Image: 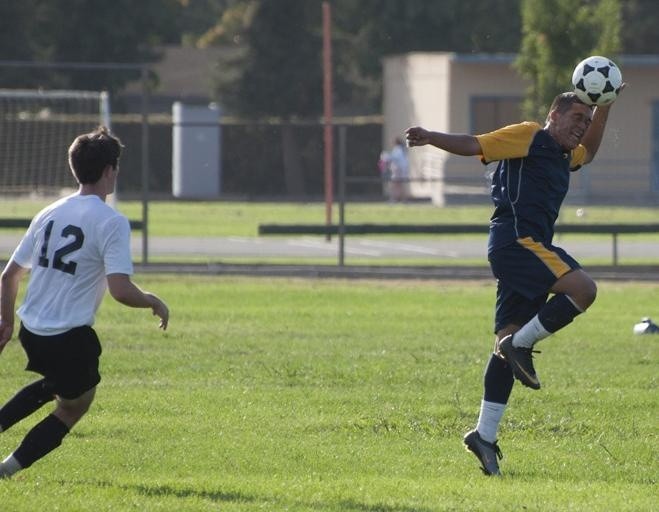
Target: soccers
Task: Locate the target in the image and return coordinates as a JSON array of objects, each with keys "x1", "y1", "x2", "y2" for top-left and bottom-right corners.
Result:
[{"x1": 572, "y1": 56, "x2": 622, "y2": 107}]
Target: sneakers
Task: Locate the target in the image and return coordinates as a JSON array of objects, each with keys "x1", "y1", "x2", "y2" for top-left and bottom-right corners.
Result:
[
  {"x1": 500, "y1": 334, "x2": 542, "y2": 391},
  {"x1": 463, "y1": 428, "x2": 501, "y2": 478}
]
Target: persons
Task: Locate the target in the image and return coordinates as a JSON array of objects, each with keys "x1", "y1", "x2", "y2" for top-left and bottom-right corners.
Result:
[
  {"x1": 385, "y1": 135, "x2": 410, "y2": 204},
  {"x1": 0, "y1": 126, "x2": 169, "y2": 481},
  {"x1": 404, "y1": 81, "x2": 628, "y2": 475}
]
